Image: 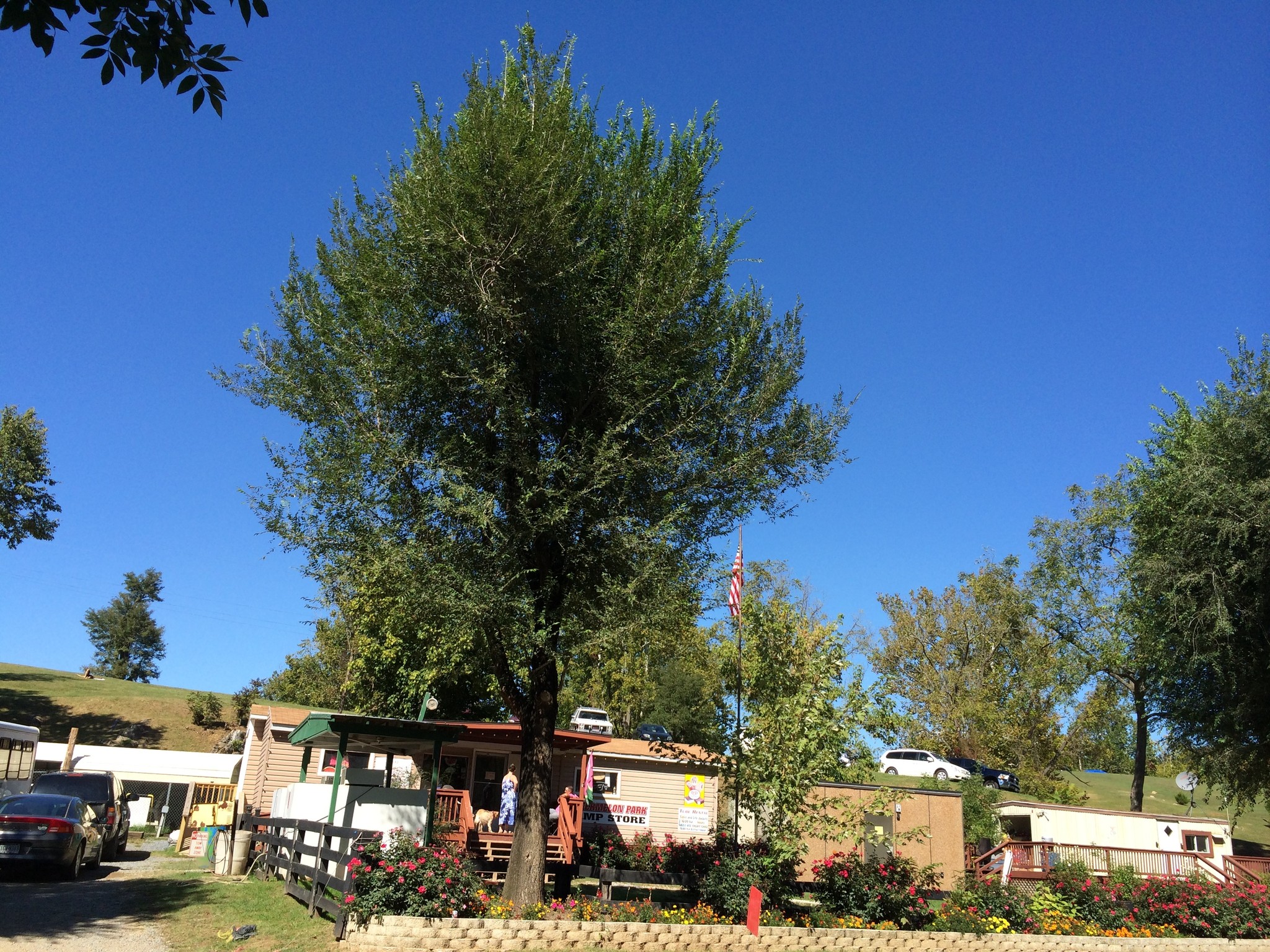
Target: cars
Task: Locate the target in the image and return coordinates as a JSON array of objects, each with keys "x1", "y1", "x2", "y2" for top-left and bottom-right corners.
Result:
[
  {"x1": 0, "y1": 793, "x2": 109, "y2": 882},
  {"x1": 631, "y1": 723, "x2": 673, "y2": 743}
]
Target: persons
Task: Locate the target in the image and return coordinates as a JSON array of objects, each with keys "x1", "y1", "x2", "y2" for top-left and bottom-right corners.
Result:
[
  {"x1": 75, "y1": 669, "x2": 103, "y2": 679},
  {"x1": 498, "y1": 764, "x2": 519, "y2": 834},
  {"x1": 549, "y1": 786, "x2": 579, "y2": 834}
]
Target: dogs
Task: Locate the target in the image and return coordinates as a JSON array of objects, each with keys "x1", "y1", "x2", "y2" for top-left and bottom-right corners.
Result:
[{"x1": 473, "y1": 808, "x2": 500, "y2": 833}]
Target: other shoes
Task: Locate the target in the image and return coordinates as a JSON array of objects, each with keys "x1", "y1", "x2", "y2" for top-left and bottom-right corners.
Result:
[
  {"x1": 503, "y1": 830, "x2": 513, "y2": 834},
  {"x1": 498, "y1": 830, "x2": 504, "y2": 833}
]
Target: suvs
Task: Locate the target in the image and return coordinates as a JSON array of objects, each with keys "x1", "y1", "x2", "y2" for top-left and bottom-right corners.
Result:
[
  {"x1": 878, "y1": 748, "x2": 971, "y2": 783},
  {"x1": 946, "y1": 757, "x2": 1020, "y2": 794},
  {"x1": 28, "y1": 770, "x2": 139, "y2": 862},
  {"x1": 569, "y1": 707, "x2": 613, "y2": 736}
]
